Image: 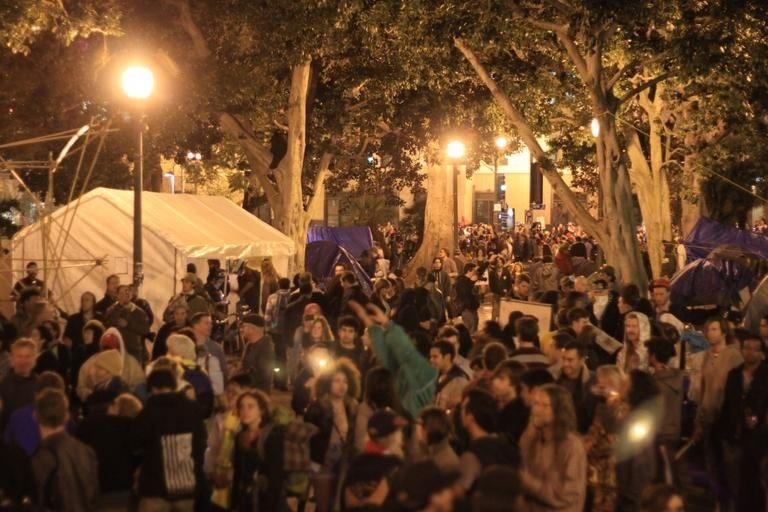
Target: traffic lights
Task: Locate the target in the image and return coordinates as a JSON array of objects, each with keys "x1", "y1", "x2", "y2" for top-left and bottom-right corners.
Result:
[{"x1": 496, "y1": 174, "x2": 505, "y2": 202}]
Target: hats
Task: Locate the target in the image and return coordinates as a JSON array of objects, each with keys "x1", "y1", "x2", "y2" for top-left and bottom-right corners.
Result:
[
  {"x1": 232, "y1": 259, "x2": 244, "y2": 274},
  {"x1": 95, "y1": 349, "x2": 123, "y2": 378},
  {"x1": 366, "y1": 408, "x2": 407, "y2": 439},
  {"x1": 649, "y1": 278, "x2": 669, "y2": 292},
  {"x1": 181, "y1": 272, "x2": 197, "y2": 284},
  {"x1": 208, "y1": 268, "x2": 224, "y2": 278},
  {"x1": 239, "y1": 314, "x2": 266, "y2": 329},
  {"x1": 299, "y1": 272, "x2": 312, "y2": 286},
  {"x1": 166, "y1": 333, "x2": 197, "y2": 361},
  {"x1": 598, "y1": 263, "x2": 616, "y2": 281}
]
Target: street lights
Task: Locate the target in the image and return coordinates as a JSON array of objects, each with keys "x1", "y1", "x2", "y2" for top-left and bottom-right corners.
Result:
[
  {"x1": 117, "y1": 64, "x2": 160, "y2": 286},
  {"x1": 184, "y1": 150, "x2": 202, "y2": 193},
  {"x1": 162, "y1": 169, "x2": 176, "y2": 194},
  {"x1": 491, "y1": 137, "x2": 506, "y2": 206},
  {"x1": 445, "y1": 139, "x2": 463, "y2": 253}
]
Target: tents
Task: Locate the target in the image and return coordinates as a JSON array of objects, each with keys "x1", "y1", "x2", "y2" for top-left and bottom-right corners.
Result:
[{"x1": 10, "y1": 188, "x2": 300, "y2": 335}]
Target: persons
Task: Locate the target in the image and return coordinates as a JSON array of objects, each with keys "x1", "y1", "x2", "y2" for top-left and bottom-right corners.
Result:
[{"x1": 1, "y1": 200, "x2": 767, "y2": 510}]
